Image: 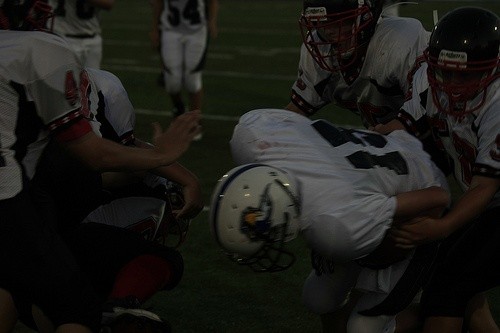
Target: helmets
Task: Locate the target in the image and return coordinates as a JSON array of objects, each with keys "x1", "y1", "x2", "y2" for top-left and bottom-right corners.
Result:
[
  {"x1": 208, "y1": 161, "x2": 301, "y2": 256},
  {"x1": 423, "y1": 5, "x2": 500, "y2": 86},
  {"x1": 104, "y1": 164, "x2": 191, "y2": 248},
  {"x1": 0, "y1": 0, "x2": 57, "y2": 33},
  {"x1": 298, "y1": 0, "x2": 383, "y2": 49}
]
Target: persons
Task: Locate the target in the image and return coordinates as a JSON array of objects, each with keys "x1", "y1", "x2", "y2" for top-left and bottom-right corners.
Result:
[
  {"x1": 284, "y1": 0, "x2": 430, "y2": 333},
  {"x1": 0, "y1": 0, "x2": 204, "y2": 333},
  {"x1": 209, "y1": 108, "x2": 451, "y2": 333},
  {"x1": 367, "y1": 6, "x2": 499, "y2": 333},
  {"x1": 152, "y1": 0, "x2": 218, "y2": 119}
]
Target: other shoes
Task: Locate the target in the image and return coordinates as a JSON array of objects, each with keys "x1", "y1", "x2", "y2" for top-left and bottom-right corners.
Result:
[{"x1": 101, "y1": 294, "x2": 170, "y2": 328}]
